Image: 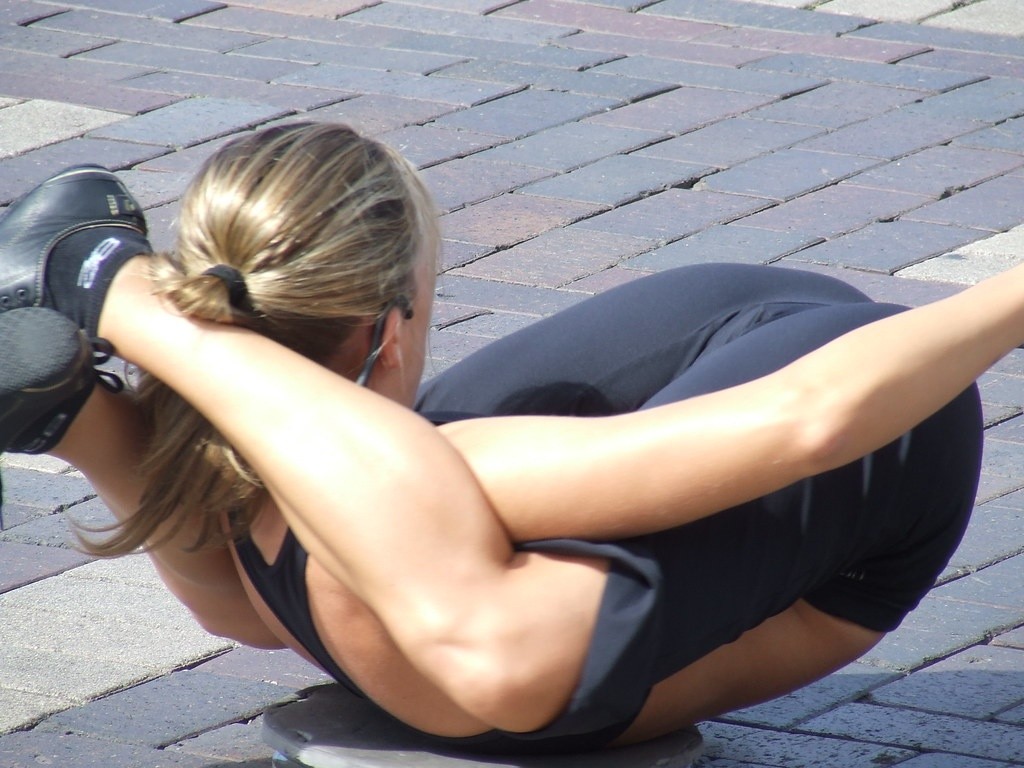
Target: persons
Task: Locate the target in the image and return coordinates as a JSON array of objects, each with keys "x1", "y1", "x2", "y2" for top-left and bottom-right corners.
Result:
[{"x1": 1, "y1": 123, "x2": 1024, "y2": 742}]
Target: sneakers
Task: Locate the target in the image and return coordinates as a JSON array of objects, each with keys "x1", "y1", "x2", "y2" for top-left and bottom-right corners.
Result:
[
  {"x1": 0, "y1": 164, "x2": 146, "y2": 312},
  {"x1": 0, "y1": 307, "x2": 97, "y2": 452}
]
